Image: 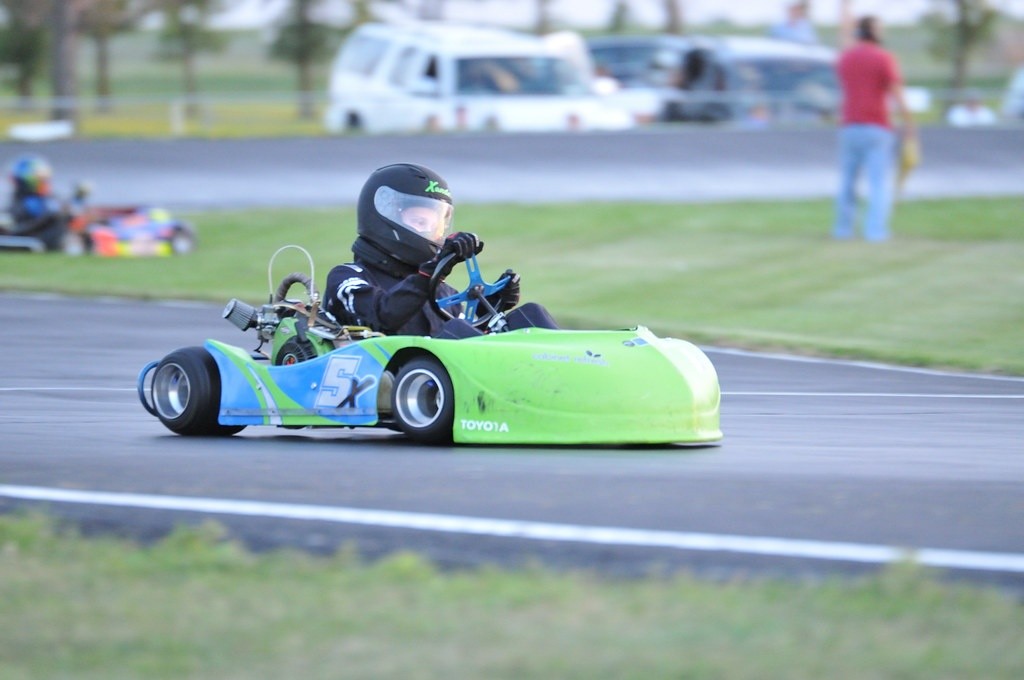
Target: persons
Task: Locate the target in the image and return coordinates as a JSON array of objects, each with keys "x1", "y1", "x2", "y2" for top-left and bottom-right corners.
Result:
[
  {"x1": 11, "y1": 155, "x2": 86, "y2": 252},
  {"x1": 656, "y1": 45, "x2": 730, "y2": 125},
  {"x1": 831, "y1": 16, "x2": 918, "y2": 243},
  {"x1": 325, "y1": 163, "x2": 520, "y2": 336}
]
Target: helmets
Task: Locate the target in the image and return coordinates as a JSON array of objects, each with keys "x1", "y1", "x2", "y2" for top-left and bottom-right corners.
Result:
[
  {"x1": 11, "y1": 156, "x2": 54, "y2": 196},
  {"x1": 352, "y1": 164, "x2": 453, "y2": 278}
]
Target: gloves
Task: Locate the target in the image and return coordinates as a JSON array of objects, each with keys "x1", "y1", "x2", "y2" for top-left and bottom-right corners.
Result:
[
  {"x1": 75, "y1": 187, "x2": 90, "y2": 198},
  {"x1": 419, "y1": 231, "x2": 484, "y2": 283},
  {"x1": 475, "y1": 269, "x2": 520, "y2": 327}
]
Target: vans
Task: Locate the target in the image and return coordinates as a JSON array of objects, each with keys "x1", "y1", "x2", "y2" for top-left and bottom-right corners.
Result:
[
  {"x1": 325, "y1": 19, "x2": 627, "y2": 133},
  {"x1": 590, "y1": 34, "x2": 854, "y2": 129}
]
